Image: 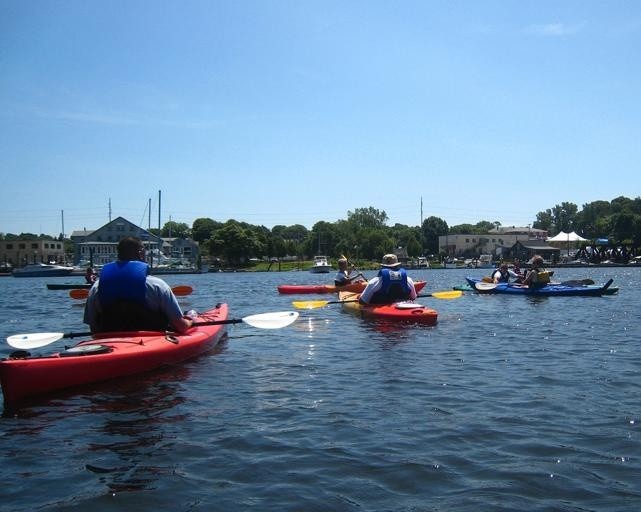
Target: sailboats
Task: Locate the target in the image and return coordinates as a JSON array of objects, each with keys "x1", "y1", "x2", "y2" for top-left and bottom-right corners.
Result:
[{"x1": 94, "y1": 186, "x2": 210, "y2": 276}]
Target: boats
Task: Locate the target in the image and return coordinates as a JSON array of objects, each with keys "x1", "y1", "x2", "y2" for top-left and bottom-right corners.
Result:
[
  {"x1": 453, "y1": 276, "x2": 619, "y2": 297},
  {"x1": 0, "y1": 302, "x2": 228, "y2": 405},
  {"x1": 12, "y1": 261, "x2": 74, "y2": 278},
  {"x1": 47, "y1": 283, "x2": 92, "y2": 290},
  {"x1": 277, "y1": 280, "x2": 438, "y2": 325},
  {"x1": 312, "y1": 256, "x2": 332, "y2": 273}
]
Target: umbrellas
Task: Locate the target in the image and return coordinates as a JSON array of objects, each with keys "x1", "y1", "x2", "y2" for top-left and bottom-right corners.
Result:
[{"x1": 545, "y1": 230, "x2": 591, "y2": 256}]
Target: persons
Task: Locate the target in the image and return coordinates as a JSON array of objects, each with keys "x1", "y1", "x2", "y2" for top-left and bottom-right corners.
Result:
[
  {"x1": 334, "y1": 259, "x2": 362, "y2": 286},
  {"x1": 83, "y1": 236, "x2": 193, "y2": 333},
  {"x1": 493, "y1": 263, "x2": 524, "y2": 283},
  {"x1": 85, "y1": 267, "x2": 96, "y2": 284},
  {"x1": 520, "y1": 255, "x2": 548, "y2": 285},
  {"x1": 356, "y1": 253, "x2": 417, "y2": 305}
]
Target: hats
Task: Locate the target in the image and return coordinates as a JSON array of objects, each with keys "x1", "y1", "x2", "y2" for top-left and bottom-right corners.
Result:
[{"x1": 379, "y1": 254, "x2": 404, "y2": 267}]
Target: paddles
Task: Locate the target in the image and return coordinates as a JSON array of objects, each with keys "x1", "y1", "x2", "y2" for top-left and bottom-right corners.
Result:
[
  {"x1": 69, "y1": 286, "x2": 193, "y2": 299},
  {"x1": 291, "y1": 291, "x2": 463, "y2": 309},
  {"x1": 6, "y1": 311, "x2": 299, "y2": 350},
  {"x1": 475, "y1": 277, "x2": 595, "y2": 290}
]
